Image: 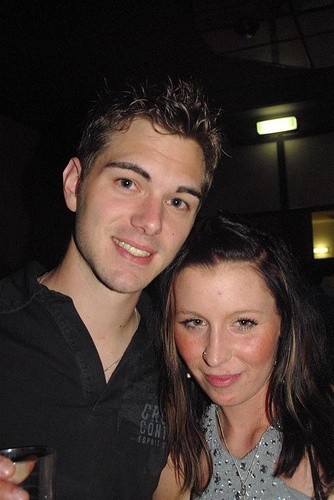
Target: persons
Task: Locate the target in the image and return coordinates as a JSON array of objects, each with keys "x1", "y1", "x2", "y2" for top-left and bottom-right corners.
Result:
[
  {"x1": 0, "y1": 74, "x2": 219, "y2": 500},
  {"x1": 151, "y1": 212, "x2": 334, "y2": 500}
]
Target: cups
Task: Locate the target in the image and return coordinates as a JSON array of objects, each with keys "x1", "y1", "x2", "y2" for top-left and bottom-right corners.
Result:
[{"x1": 0, "y1": 445, "x2": 57, "y2": 500}]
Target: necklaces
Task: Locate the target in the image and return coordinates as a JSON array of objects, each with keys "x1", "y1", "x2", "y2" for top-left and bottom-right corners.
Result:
[
  {"x1": 215, "y1": 405, "x2": 278, "y2": 500},
  {"x1": 39, "y1": 270, "x2": 139, "y2": 374}
]
